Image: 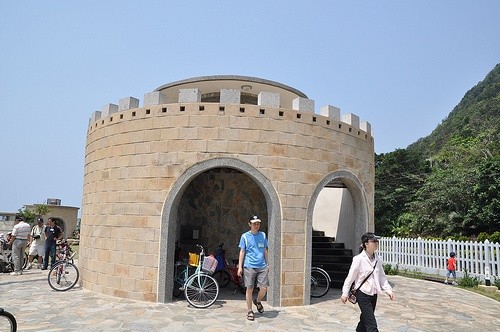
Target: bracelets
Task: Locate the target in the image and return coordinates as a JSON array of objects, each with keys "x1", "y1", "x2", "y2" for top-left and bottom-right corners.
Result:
[{"x1": 266, "y1": 263, "x2": 269, "y2": 266}]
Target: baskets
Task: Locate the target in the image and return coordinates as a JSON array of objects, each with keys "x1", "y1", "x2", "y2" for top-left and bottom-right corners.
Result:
[{"x1": 202, "y1": 255, "x2": 218, "y2": 275}]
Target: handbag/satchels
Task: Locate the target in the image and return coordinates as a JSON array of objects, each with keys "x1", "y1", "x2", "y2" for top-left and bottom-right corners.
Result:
[{"x1": 348, "y1": 289, "x2": 357, "y2": 304}]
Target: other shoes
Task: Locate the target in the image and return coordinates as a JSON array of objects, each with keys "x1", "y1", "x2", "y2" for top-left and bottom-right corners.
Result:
[
  {"x1": 452, "y1": 281, "x2": 459, "y2": 285},
  {"x1": 41, "y1": 265, "x2": 47, "y2": 270},
  {"x1": 25, "y1": 265, "x2": 33, "y2": 270},
  {"x1": 445, "y1": 281, "x2": 449, "y2": 284},
  {"x1": 10, "y1": 271, "x2": 22, "y2": 275}
]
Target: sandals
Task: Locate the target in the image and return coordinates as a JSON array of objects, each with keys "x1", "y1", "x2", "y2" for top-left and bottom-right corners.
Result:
[
  {"x1": 253, "y1": 298, "x2": 264, "y2": 313},
  {"x1": 247, "y1": 311, "x2": 254, "y2": 320}
]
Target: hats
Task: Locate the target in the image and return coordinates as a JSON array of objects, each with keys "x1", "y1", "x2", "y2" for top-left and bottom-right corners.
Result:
[
  {"x1": 362, "y1": 232, "x2": 381, "y2": 243},
  {"x1": 450, "y1": 252, "x2": 455, "y2": 257},
  {"x1": 249, "y1": 215, "x2": 262, "y2": 223},
  {"x1": 36, "y1": 217, "x2": 43, "y2": 223}
]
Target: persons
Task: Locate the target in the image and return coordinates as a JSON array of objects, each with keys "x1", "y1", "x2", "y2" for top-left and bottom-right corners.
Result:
[
  {"x1": 0, "y1": 232, "x2": 12, "y2": 250},
  {"x1": 72, "y1": 230, "x2": 76, "y2": 236},
  {"x1": 25, "y1": 218, "x2": 46, "y2": 270},
  {"x1": 237, "y1": 214, "x2": 269, "y2": 320},
  {"x1": 6, "y1": 215, "x2": 31, "y2": 276},
  {"x1": 41, "y1": 217, "x2": 65, "y2": 270},
  {"x1": 341, "y1": 232, "x2": 395, "y2": 332},
  {"x1": 444, "y1": 252, "x2": 458, "y2": 285}
]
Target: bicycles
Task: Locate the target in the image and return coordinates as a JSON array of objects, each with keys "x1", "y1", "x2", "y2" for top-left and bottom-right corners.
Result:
[
  {"x1": 174, "y1": 239, "x2": 246, "y2": 309},
  {"x1": 310, "y1": 264, "x2": 332, "y2": 299},
  {"x1": 47, "y1": 237, "x2": 79, "y2": 292},
  {"x1": 0, "y1": 238, "x2": 28, "y2": 272}
]
